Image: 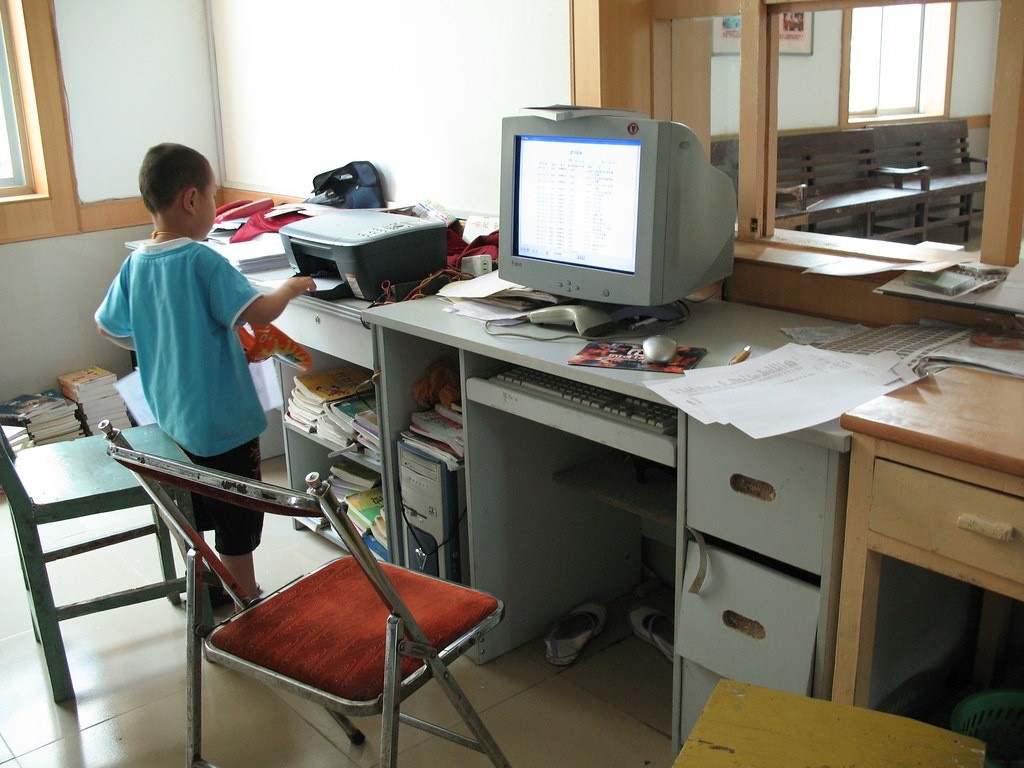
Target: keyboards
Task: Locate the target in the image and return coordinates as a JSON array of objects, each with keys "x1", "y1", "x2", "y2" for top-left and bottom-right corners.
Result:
[{"x1": 489, "y1": 367, "x2": 678, "y2": 434}]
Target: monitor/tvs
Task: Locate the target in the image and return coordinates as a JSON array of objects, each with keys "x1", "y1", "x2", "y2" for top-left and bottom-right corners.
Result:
[{"x1": 498, "y1": 114, "x2": 738, "y2": 339}]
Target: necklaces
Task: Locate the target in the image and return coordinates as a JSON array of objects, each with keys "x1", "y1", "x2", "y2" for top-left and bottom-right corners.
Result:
[{"x1": 152, "y1": 231, "x2": 182, "y2": 239}]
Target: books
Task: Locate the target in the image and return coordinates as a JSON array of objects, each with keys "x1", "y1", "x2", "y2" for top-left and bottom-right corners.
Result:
[
  {"x1": 284, "y1": 367, "x2": 388, "y2": 561},
  {"x1": 400, "y1": 402, "x2": 464, "y2": 472},
  {"x1": 0, "y1": 366, "x2": 132, "y2": 501},
  {"x1": 437, "y1": 279, "x2": 574, "y2": 313}
]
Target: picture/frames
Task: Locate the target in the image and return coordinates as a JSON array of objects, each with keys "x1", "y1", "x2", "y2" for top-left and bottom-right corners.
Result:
[{"x1": 711, "y1": 11, "x2": 815, "y2": 57}]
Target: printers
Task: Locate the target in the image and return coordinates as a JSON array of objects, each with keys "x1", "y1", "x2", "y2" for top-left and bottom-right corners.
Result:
[{"x1": 279, "y1": 209, "x2": 448, "y2": 302}]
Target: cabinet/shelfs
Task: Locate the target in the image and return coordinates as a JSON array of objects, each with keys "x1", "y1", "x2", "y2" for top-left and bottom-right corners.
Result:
[
  {"x1": 830, "y1": 338, "x2": 1024, "y2": 709},
  {"x1": 359, "y1": 285, "x2": 949, "y2": 768},
  {"x1": 124, "y1": 237, "x2": 394, "y2": 568}
]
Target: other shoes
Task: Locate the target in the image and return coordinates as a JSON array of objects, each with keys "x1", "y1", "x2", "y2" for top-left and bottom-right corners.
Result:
[
  {"x1": 543, "y1": 601, "x2": 607, "y2": 666},
  {"x1": 209, "y1": 580, "x2": 260, "y2": 607},
  {"x1": 630, "y1": 607, "x2": 673, "y2": 662}
]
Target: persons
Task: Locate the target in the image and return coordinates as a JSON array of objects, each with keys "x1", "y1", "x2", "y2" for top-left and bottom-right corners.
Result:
[{"x1": 95, "y1": 142, "x2": 317, "y2": 615}]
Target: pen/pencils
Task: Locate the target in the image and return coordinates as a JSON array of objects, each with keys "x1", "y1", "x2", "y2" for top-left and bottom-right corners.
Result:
[
  {"x1": 628, "y1": 317, "x2": 658, "y2": 331},
  {"x1": 725, "y1": 344, "x2": 751, "y2": 369}
]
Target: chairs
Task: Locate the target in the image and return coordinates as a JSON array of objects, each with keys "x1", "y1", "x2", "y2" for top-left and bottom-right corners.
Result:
[
  {"x1": 100, "y1": 419, "x2": 508, "y2": 768},
  {"x1": 0, "y1": 419, "x2": 215, "y2": 705},
  {"x1": 670, "y1": 679, "x2": 987, "y2": 768}
]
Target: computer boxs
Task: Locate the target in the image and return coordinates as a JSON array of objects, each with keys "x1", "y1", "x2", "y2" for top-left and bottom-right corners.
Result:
[{"x1": 396, "y1": 441, "x2": 470, "y2": 587}]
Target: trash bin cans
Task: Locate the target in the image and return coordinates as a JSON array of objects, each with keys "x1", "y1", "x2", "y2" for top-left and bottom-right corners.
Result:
[{"x1": 949, "y1": 690, "x2": 1024, "y2": 768}]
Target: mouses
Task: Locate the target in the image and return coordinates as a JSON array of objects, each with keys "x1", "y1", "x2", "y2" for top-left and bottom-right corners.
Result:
[{"x1": 643, "y1": 334, "x2": 678, "y2": 364}]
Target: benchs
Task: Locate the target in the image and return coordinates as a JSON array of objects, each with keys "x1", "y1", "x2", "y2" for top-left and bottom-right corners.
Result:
[
  {"x1": 711, "y1": 128, "x2": 929, "y2": 245},
  {"x1": 866, "y1": 120, "x2": 988, "y2": 243}
]
